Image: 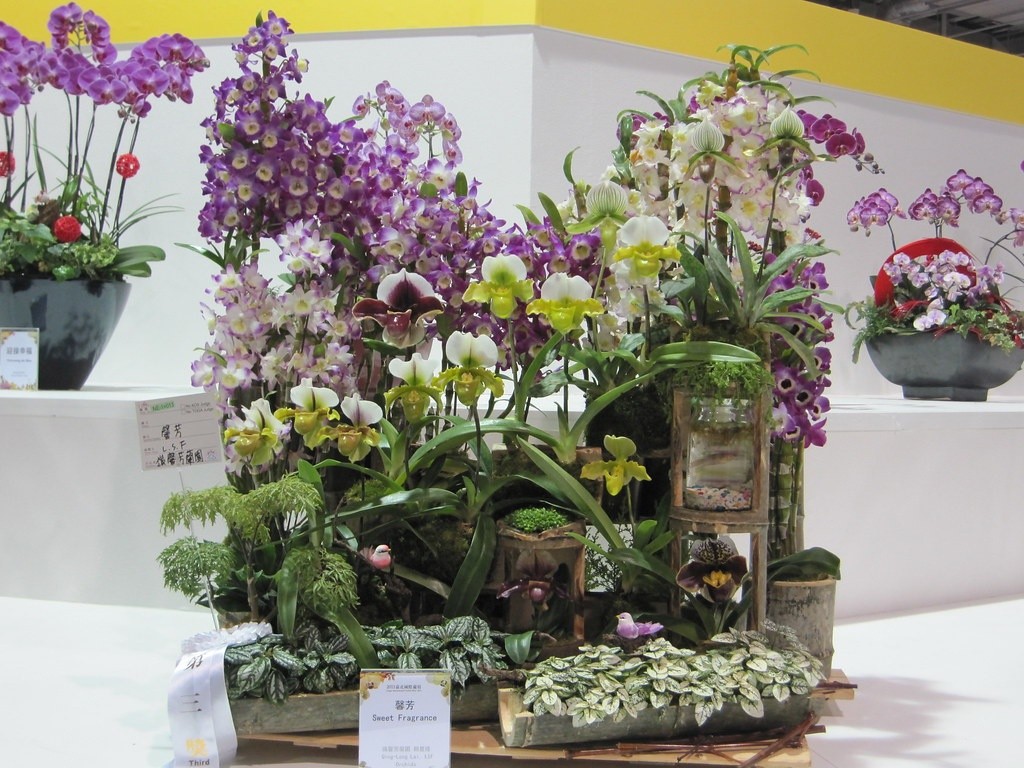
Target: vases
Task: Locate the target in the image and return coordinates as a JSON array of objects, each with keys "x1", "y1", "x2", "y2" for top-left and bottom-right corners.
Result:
[
  {"x1": 0, "y1": 276, "x2": 131, "y2": 389},
  {"x1": 863, "y1": 329, "x2": 1024, "y2": 402},
  {"x1": 742, "y1": 570, "x2": 837, "y2": 680}
]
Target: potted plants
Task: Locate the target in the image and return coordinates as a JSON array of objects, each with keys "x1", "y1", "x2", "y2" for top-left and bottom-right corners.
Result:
[
  {"x1": 494, "y1": 506, "x2": 588, "y2": 646},
  {"x1": 497, "y1": 637, "x2": 816, "y2": 755},
  {"x1": 220, "y1": 609, "x2": 512, "y2": 738}
]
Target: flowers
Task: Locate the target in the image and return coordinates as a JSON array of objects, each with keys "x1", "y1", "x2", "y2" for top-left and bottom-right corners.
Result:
[
  {"x1": 152, "y1": 4, "x2": 887, "y2": 610},
  {"x1": 0, "y1": 0, "x2": 211, "y2": 291},
  {"x1": 842, "y1": 167, "x2": 1024, "y2": 366}
]
